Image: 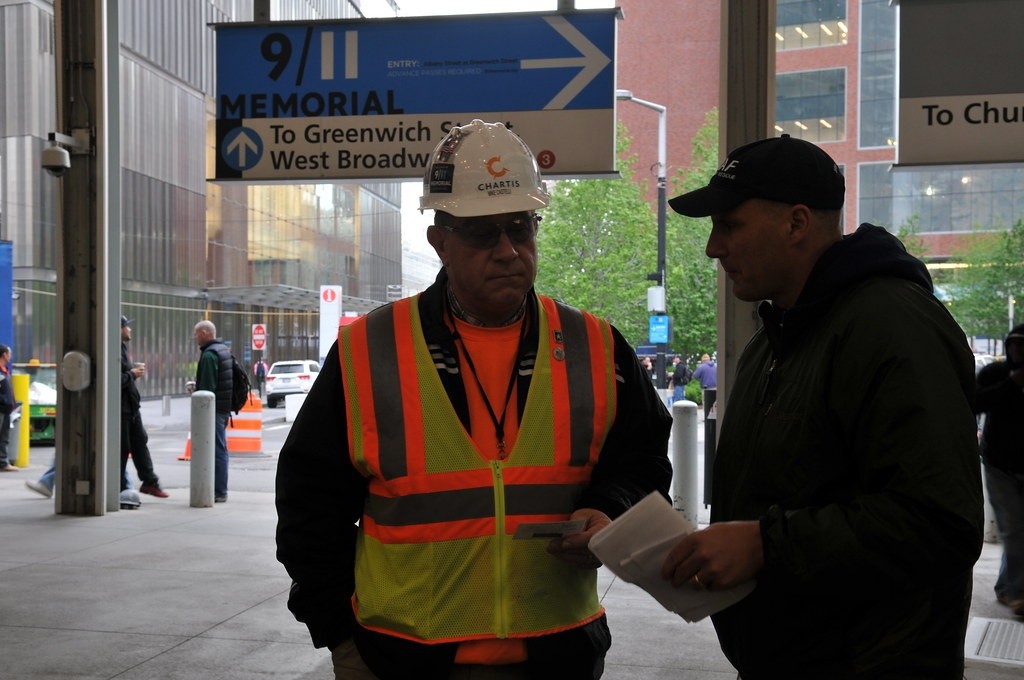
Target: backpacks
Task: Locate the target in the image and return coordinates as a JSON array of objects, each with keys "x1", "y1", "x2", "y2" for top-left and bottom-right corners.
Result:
[{"x1": 230, "y1": 354, "x2": 253, "y2": 415}]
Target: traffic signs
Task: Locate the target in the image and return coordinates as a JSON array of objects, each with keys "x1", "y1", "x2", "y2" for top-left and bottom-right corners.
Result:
[{"x1": 201, "y1": 6, "x2": 628, "y2": 188}]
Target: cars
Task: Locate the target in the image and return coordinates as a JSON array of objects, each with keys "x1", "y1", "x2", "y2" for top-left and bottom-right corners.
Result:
[{"x1": 9, "y1": 359, "x2": 56, "y2": 445}]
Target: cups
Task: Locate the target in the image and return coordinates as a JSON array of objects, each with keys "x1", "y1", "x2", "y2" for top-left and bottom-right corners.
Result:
[
  {"x1": 134, "y1": 363, "x2": 145, "y2": 376},
  {"x1": 186, "y1": 382, "x2": 196, "y2": 394}
]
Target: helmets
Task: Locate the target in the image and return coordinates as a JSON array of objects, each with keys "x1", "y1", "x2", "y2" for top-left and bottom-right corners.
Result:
[{"x1": 418, "y1": 117, "x2": 550, "y2": 220}]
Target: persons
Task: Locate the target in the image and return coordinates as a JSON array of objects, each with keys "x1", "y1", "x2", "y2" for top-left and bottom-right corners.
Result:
[
  {"x1": 671, "y1": 355, "x2": 686, "y2": 403},
  {"x1": 253, "y1": 357, "x2": 269, "y2": 398},
  {"x1": 660, "y1": 135, "x2": 983, "y2": 680},
  {"x1": 23, "y1": 462, "x2": 56, "y2": 497},
  {"x1": 641, "y1": 356, "x2": 652, "y2": 381},
  {"x1": 972, "y1": 324, "x2": 1024, "y2": 626},
  {"x1": 120, "y1": 314, "x2": 170, "y2": 498},
  {"x1": 272, "y1": 119, "x2": 673, "y2": 680},
  {"x1": 192, "y1": 319, "x2": 233, "y2": 502},
  {"x1": 0, "y1": 344, "x2": 20, "y2": 472},
  {"x1": 693, "y1": 353, "x2": 717, "y2": 422}
]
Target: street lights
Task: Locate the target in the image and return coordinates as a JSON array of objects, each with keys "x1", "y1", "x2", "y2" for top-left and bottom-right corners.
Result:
[{"x1": 616, "y1": 88, "x2": 671, "y2": 388}]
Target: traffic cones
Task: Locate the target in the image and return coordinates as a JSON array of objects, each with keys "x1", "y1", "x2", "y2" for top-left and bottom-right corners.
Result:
[{"x1": 175, "y1": 430, "x2": 192, "y2": 461}]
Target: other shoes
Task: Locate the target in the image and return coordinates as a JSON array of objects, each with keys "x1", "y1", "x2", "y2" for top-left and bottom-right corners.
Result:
[
  {"x1": 2, "y1": 463, "x2": 19, "y2": 471},
  {"x1": 25, "y1": 478, "x2": 53, "y2": 498}
]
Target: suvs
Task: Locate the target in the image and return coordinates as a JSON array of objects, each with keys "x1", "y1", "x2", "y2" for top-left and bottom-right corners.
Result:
[{"x1": 264, "y1": 359, "x2": 321, "y2": 408}]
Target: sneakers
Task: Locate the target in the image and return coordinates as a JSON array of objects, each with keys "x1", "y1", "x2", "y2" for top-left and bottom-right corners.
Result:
[
  {"x1": 214, "y1": 492, "x2": 228, "y2": 503},
  {"x1": 139, "y1": 483, "x2": 168, "y2": 497}
]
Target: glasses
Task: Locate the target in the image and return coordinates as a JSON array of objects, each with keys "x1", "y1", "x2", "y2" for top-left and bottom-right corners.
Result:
[{"x1": 438, "y1": 211, "x2": 538, "y2": 250}]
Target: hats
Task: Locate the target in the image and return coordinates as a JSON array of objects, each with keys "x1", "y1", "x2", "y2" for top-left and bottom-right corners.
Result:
[
  {"x1": 120, "y1": 315, "x2": 135, "y2": 328},
  {"x1": 668, "y1": 133, "x2": 845, "y2": 218}
]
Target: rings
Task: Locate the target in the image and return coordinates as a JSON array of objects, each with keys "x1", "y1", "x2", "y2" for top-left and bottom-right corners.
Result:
[
  {"x1": 694, "y1": 575, "x2": 704, "y2": 587},
  {"x1": 588, "y1": 554, "x2": 595, "y2": 565}
]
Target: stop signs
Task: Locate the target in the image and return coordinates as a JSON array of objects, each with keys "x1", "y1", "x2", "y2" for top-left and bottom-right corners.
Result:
[{"x1": 250, "y1": 323, "x2": 267, "y2": 351}]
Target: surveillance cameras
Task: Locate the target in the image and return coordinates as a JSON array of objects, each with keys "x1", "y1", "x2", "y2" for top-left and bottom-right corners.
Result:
[{"x1": 41, "y1": 146, "x2": 71, "y2": 177}]
